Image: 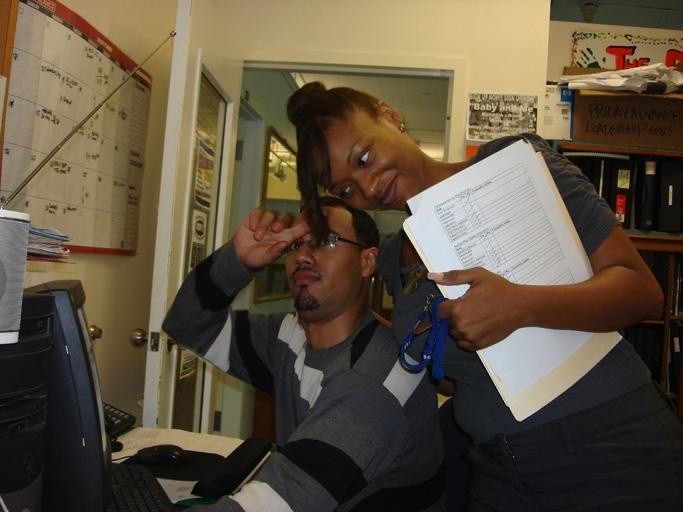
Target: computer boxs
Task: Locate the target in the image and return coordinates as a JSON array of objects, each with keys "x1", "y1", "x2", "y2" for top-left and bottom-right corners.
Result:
[{"x1": 0, "y1": 292, "x2": 56, "y2": 512}]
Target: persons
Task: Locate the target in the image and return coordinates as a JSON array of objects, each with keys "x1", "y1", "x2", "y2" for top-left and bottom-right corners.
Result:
[
  {"x1": 285, "y1": 79, "x2": 683, "y2": 512},
  {"x1": 160, "y1": 193, "x2": 449, "y2": 511}
]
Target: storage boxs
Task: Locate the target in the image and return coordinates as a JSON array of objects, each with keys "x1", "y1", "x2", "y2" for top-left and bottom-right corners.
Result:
[{"x1": 573, "y1": 89, "x2": 683, "y2": 151}]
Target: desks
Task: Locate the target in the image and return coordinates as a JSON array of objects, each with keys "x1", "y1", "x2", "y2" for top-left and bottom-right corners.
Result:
[{"x1": 111, "y1": 427, "x2": 277, "y2": 505}]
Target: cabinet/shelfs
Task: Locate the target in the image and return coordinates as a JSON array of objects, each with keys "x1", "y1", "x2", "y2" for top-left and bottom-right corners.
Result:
[{"x1": 546, "y1": 139, "x2": 683, "y2": 416}]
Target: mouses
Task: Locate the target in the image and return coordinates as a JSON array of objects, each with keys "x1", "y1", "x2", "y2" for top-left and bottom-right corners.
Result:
[{"x1": 137, "y1": 445, "x2": 187, "y2": 467}]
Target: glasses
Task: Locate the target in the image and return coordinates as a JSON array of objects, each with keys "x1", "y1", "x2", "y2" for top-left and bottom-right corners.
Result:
[{"x1": 285, "y1": 227, "x2": 370, "y2": 255}]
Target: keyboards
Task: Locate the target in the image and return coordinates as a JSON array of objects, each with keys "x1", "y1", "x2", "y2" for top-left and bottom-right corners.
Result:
[{"x1": 110, "y1": 463, "x2": 176, "y2": 512}]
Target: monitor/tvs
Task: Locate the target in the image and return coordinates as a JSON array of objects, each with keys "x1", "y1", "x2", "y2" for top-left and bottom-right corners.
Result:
[{"x1": 23, "y1": 280, "x2": 113, "y2": 512}]
